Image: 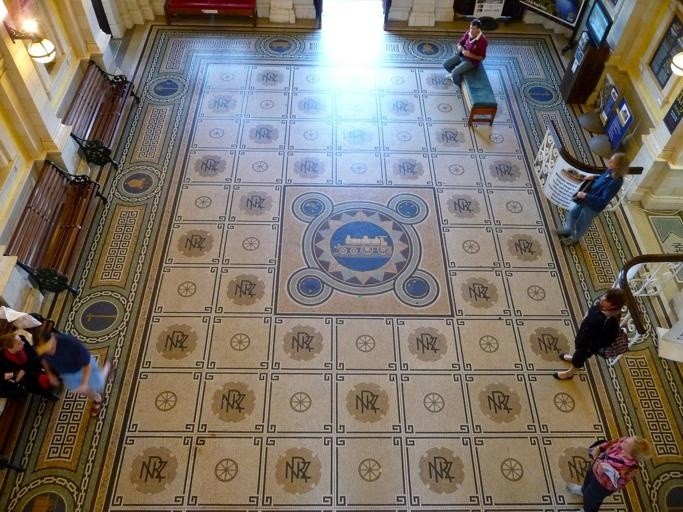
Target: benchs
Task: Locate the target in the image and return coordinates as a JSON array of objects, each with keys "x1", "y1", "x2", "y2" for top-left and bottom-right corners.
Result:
[
  {"x1": 455, "y1": 51, "x2": 497, "y2": 126},
  {"x1": 62, "y1": 61, "x2": 140, "y2": 171},
  {"x1": 0, "y1": 297, "x2": 59, "y2": 486},
  {"x1": 4, "y1": 159, "x2": 108, "y2": 295},
  {"x1": 164, "y1": 0, "x2": 258, "y2": 28}
]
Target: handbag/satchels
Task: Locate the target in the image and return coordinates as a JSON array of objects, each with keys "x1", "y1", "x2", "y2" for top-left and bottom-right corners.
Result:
[
  {"x1": 589, "y1": 440, "x2": 609, "y2": 459},
  {"x1": 598, "y1": 315, "x2": 628, "y2": 359},
  {"x1": 572, "y1": 179, "x2": 595, "y2": 205}
]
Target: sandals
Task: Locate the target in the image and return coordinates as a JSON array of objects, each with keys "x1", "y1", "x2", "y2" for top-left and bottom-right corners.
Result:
[{"x1": 89, "y1": 393, "x2": 104, "y2": 417}]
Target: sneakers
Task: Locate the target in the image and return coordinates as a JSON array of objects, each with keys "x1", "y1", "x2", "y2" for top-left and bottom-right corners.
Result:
[
  {"x1": 560, "y1": 237, "x2": 574, "y2": 246},
  {"x1": 557, "y1": 228, "x2": 567, "y2": 235},
  {"x1": 567, "y1": 483, "x2": 583, "y2": 496}
]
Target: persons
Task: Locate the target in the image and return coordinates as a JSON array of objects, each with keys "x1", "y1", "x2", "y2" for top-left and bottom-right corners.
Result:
[
  {"x1": 553, "y1": 288, "x2": 627, "y2": 380},
  {"x1": 0, "y1": 333, "x2": 61, "y2": 402},
  {"x1": 442, "y1": 18, "x2": 488, "y2": 86},
  {"x1": 31, "y1": 329, "x2": 112, "y2": 418},
  {"x1": 566, "y1": 435, "x2": 654, "y2": 512},
  {"x1": 553, "y1": 152, "x2": 630, "y2": 246}
]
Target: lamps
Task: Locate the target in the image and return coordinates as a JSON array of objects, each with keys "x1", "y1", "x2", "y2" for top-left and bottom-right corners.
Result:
[
  {"x1": 3, "y1": 21, "x2": 55, "y2": 64},
  {"x1": 671, "y1": 52, "x2": 683, "y2": 77}
]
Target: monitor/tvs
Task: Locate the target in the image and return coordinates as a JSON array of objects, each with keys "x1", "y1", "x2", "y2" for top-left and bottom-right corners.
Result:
[{"x1": 586, "y1": 0, "x2": 613, "y2": 49}]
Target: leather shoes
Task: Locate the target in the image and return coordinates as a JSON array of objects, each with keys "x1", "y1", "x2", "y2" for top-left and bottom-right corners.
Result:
[
  {"x1": 559, "y1": 353, "x2": 572, "y2": 362},
  {"x1": 553, "y1": 372, "x2": 573, "y2": 380}
]
